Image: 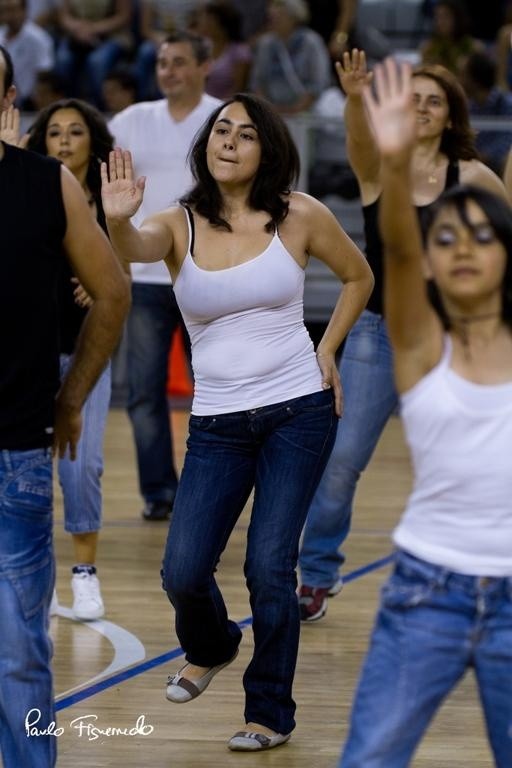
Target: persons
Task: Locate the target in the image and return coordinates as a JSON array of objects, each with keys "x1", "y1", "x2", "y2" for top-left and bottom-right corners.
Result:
[{"x1": 1, "y1": 3, "x2": 506, "y2": 766}]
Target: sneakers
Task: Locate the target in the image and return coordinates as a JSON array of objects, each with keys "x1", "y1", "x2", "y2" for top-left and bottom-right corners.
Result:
[
  {"x1": 141, "y1": 499, "x2": 173, "y2": 520},
  {"x1": 68, "y1": 565, "x2": 106, "y2": 621},
  {"x1": 295, "y1": 574, "x2": 345, "y2": 623}
]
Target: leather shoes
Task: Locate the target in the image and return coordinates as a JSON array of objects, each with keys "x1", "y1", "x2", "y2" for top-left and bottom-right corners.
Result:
[
  {"x1": 225, "y1": 729, "x2": 294, "y2": 752},
  {"x1": 163, "y1": 645, "x2": 240, "y2": 705}
]
